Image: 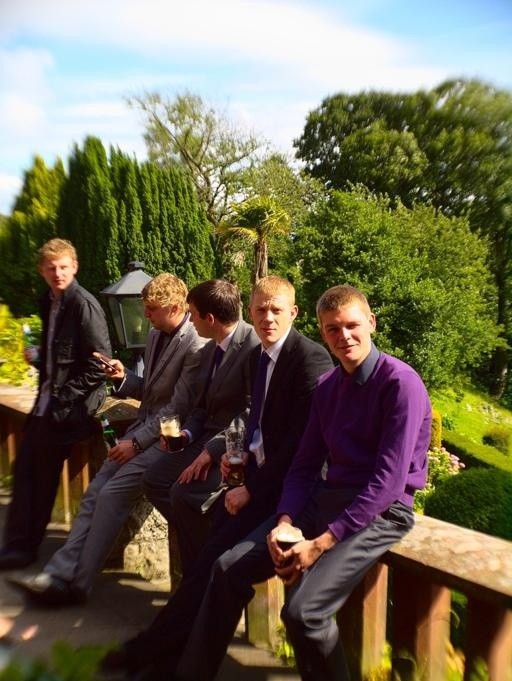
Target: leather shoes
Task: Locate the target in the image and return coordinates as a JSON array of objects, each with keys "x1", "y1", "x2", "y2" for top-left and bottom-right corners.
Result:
[{"x1": 6, "y1": 576, "x2": 87, "y2": 613}]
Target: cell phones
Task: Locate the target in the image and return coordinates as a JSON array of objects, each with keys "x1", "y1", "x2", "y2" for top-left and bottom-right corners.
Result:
[{"x1": 98, "y1": 359, "x2": 116, "y2": 372}]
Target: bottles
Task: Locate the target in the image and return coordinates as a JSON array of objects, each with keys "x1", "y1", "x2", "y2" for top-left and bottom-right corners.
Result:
[
  {"x1": 18, "y1": 318, "x2": 39, "y2": 367},
  {"x1": 97, "y1": 415, "x2": 119, "y2": 457}
]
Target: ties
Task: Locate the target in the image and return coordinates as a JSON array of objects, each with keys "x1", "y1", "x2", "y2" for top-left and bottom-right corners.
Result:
[
  {"x1": 243, "y1": 352, "x2": 271, "y2": 451},
  {"x1": 210, "y1": 345, "x2": 225, "y2": 371}
]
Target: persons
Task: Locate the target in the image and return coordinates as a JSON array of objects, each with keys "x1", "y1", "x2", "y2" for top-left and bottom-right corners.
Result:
[
  {"x1": 0, "y1": 237, "x2": 112, "y2": 572},
  {"x1": 99, "y1": 275, "x2": 335, "y2": 681},
  {"x1": 172, "y1": 283, "x2": 432, "y2": 681},
  {"x1": 139, "y1": 279, "x2": 263, "y2": 578},
  {"x1": 4, "y1": 272, "x2": 212, "y2": 607}
]
